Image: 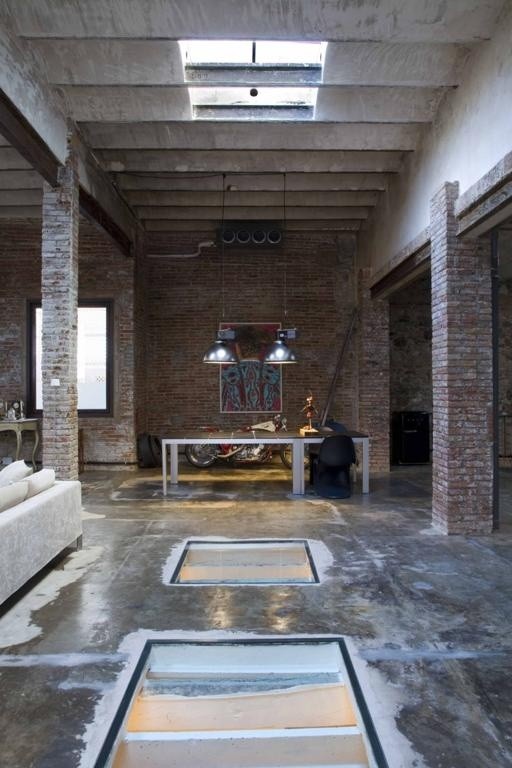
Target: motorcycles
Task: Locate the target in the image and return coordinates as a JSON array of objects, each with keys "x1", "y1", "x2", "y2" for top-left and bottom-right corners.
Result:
[{"x1": 186, "y1": 414, "x2": 310, "y2": 470}]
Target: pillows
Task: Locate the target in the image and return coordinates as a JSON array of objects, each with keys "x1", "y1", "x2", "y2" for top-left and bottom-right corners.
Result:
[
  {"x1": 0, "y1": 480, "x2": 28, "y2": 513},
  {"x1": 24, "y1": 469, "x2": 56, "y2": 498},
  {"x1": 0, "y1": 459, "x2": 33, "y2": 488}
]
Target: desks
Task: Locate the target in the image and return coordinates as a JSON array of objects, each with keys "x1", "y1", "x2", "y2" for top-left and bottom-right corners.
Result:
[{"x1": 0, "y1": 418, "x2": 39, "y2": 469}]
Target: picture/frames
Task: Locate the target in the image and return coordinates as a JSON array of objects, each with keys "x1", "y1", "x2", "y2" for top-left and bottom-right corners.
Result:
[{"x1": 218, "y1": 322, "x2": 282, "y2": 414}]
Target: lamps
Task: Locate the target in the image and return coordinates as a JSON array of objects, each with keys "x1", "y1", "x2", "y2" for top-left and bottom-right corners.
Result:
[
  {"x1": 202, "y1": 173, "x2": 239, "y2": 365},
  {"x1": 263, "y1": 173, "x2": 299, "y2": 364},
  {"x1": 161, "y1": 430, "x2": 372, "y2": 495}
]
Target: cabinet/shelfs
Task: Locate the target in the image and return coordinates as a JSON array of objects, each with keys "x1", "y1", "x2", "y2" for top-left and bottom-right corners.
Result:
[{"x1": 392, "y1": 411, "x2": 431, "y2": 465}]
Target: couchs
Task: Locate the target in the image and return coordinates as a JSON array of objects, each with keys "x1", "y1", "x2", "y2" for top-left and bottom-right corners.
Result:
[{"x1": 0, "y1": 479, "x2": 83, "y2": 606}]
